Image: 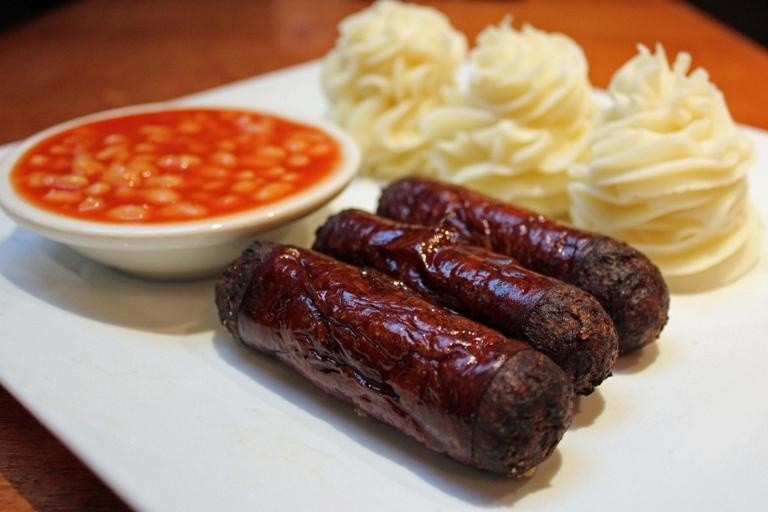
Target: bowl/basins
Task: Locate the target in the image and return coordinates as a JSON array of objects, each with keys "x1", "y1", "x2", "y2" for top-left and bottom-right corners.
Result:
[{"x1": 0, "y1": 101, "x2": 362, "y2": 285}]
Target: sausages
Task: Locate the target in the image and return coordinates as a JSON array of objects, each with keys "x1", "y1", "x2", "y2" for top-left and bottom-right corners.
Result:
[
  {"x1": 216, "y1": 239, "x2": 574, "y2": 479},
  {"x1": 375, "y1": 176, "x2": 670, "y2": 356},
  {"x1": 310, "y1": 209, "x2": 619, "y2": 396}
]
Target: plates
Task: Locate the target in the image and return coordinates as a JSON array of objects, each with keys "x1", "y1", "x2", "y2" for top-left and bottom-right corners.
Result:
[{"x1": 0, "y1": 53, "x2": 766, "y2": 511}]
